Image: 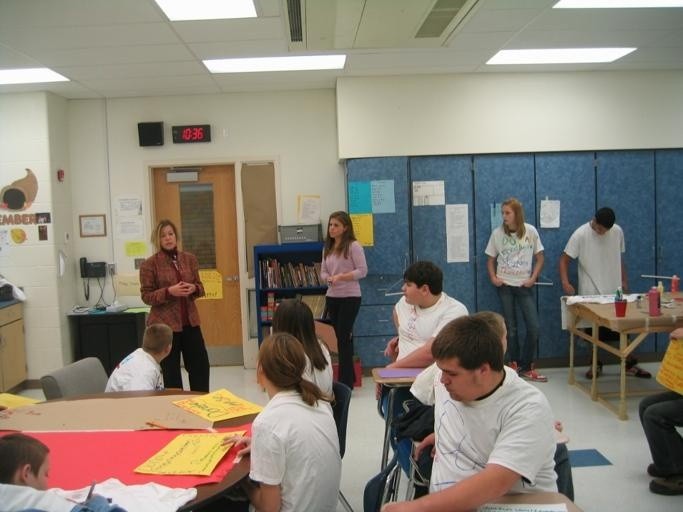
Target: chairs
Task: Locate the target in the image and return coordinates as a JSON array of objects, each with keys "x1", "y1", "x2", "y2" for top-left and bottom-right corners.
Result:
[{"x1": 40, "y1": 355, "x2": 110, "y2": 398}]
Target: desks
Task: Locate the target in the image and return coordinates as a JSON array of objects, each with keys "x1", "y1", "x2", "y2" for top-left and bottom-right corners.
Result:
[
  {"x1": 1, "y1": 390, "x2": 269, "y2": 511},
  {"x1": 331, "y1": 361, "x2": 585, "y2": 512},
  {"x1": 560, "y1": 291, "x2": 681, "y2": 421}
]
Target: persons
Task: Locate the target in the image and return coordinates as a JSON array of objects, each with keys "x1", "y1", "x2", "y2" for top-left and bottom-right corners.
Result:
[
  {"x1": 406, "y1": 308, "x2": 563, "y2": 435},
  {"x1": 0, "y1": 430, "x2": 123, "y2": 510},
  {"x1": 220, "y1": 331, "x2": 341, "y2": 511},
  {"x1": 269, "y1": 297, "x2": 322, "y2": 406},
  {"x1": 558, "y1": 204, "x2": 652, "y2": 380},
  {"x1": 138, "y1": 219, "x2": 209, "y2": 393},
  {"x1": 375, "y1": 315, "x2": 561, "y2": 511},
  {"x1": 100, "y1": 323, "x2": 173, "y2": 396},
  {"x1": 317, "y1": 207, "x2": 368, "y2": 392},
  {"x1": 373, "y1": 260, "x2": 469, "y2": 421},
  {"x1": 482, "y1": 196, "x2": 550, "y2": 384},
  {"x1": 637, "y1": 324, "x2": 683, "y2": 496}
]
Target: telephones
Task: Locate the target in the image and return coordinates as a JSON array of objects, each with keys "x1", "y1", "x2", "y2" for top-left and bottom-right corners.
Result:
[{"x1": 80, "y1": 257, "x2": 106, "y2": 277}]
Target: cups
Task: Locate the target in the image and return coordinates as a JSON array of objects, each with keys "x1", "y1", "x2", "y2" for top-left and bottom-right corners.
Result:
[{"x1": 615, "y1": 299, "x2": 627, "y2": 318}]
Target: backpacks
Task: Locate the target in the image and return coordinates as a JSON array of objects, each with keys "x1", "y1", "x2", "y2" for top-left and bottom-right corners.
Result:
[{"x1": 391, "y1": 400, "x2": 434, "y2": 440}]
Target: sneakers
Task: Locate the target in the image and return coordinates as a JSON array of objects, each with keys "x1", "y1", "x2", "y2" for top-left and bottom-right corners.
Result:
[
  {"x1": 625, "y1": 367, "x2": 651, "y2": 378},
  {"x1": 522, "y1": 370, "x2": 546, "y2": 382},
  {"x1": 649, "y1": 477, "x2": 682, "y2": 495},
  {"x1": 585, "y1": 364, "x2": 601, "y2": 379},
  {"x1": 646, "y1": 463, "x2": 664, "y2": 477},
  {"x1": 509, "y1": 361, "x2": 518, "y2": 374}
]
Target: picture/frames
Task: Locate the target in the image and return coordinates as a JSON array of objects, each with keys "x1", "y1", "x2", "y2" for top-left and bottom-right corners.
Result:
[{"x1": 77, "y1": 214, "x2": 107, "y2": 239}]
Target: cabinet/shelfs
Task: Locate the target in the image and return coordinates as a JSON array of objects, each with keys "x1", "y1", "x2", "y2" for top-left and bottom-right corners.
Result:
[
  {"x1": 253, "y1": 241, "x2": 335, "y2": 349},
  {"x1": 0, "y1": 303, "x2": 28, "y2": 393},
  {"x1": 65, "y1": 312, "x2": 145, "y2": 377}
]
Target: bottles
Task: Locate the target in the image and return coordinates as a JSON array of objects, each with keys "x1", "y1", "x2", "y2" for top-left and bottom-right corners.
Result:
[
  {"x1": 672, "y1": 275, "x2": 679, "y2": 294},
  {"x1": 657, "y1": 281, "x2": 664, "y2": 299},
  {"x1": 636, "y1": 295, "x2": 643, "y2": 309}
]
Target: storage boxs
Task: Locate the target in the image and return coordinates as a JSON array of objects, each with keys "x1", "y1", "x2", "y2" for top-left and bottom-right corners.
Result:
[{"x1": 276, "y1": 223, "x2": 323, "y2": 246}]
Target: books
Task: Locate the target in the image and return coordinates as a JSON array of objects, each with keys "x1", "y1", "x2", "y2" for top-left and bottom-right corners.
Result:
[
  {"x1": 257, "y1": 258, "x2": 327, "y2": 289},
  {"x1": 258, "y1": 292, "x2": 301, "y2": 322}
]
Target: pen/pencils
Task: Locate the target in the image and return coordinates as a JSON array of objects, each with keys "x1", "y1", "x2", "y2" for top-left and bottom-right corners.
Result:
[
  {"x1": 146, "y1": 422, "x2": 168, "y2": 429},
  {"x1": 615, "y1": 286, "x2": 626, "y2": 301},
  {"x1": 87, "y1": 480, "x2": 97, "y2": 499}
]
